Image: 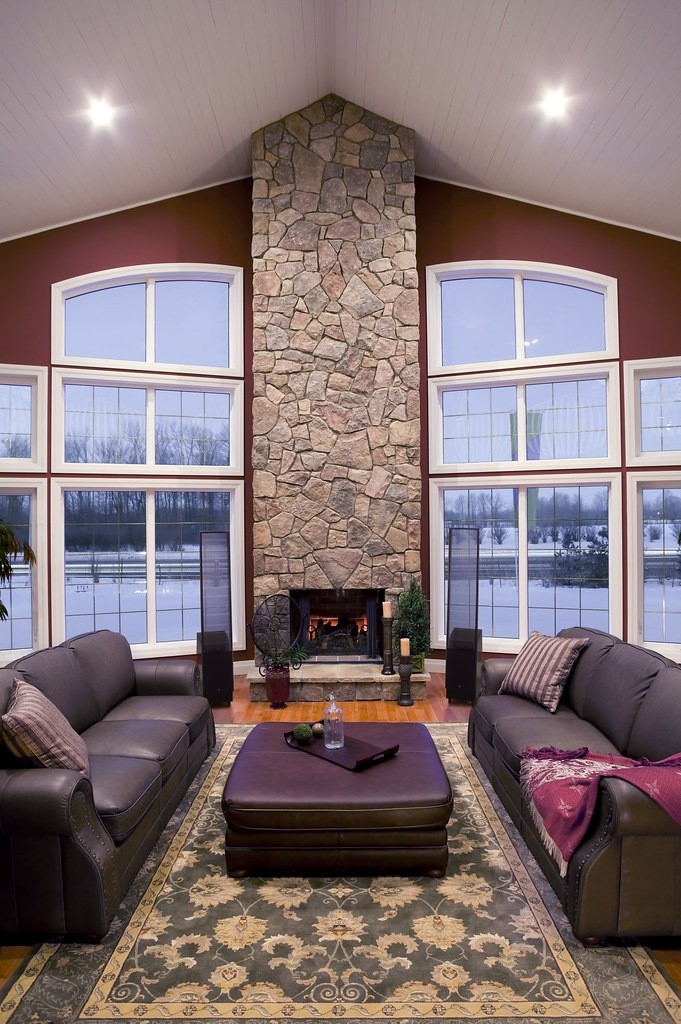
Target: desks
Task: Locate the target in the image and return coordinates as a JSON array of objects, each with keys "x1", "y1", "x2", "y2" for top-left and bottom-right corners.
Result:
[{"x1": 221, "y1": 721, "x2": 455, "y2": 881}]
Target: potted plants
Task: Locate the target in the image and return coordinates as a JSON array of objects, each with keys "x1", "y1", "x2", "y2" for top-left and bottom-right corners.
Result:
[
  {"x1": 393, "y1": 574, "x2": 430, "y2": 673},
  {"x1": 264, "y1": 644, "x2": 310, "y2": 710}
]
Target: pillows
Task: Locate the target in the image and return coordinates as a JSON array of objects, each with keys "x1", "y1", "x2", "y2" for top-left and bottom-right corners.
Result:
[
  {"x1": 2, "y1": 677, "x2": 88, "y2": 783},
  {"x1": 498, "y1": 630, "x2": 589, "y2": 715}
]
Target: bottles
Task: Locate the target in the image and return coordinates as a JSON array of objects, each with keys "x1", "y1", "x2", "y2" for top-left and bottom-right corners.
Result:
[{"x1": 325, "y1": 693, "x2": 344, "y2": 749}]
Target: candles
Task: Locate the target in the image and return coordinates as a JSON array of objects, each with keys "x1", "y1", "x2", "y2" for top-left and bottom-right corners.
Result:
[
  {"x1": 382, "y1": 601, "x2": 392, "y2": 618},
  {"x1": 400, "y1": 637, "x2": 409, "y2": 656}
]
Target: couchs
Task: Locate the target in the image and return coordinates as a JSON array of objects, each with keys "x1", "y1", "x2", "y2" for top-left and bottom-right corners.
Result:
[
  {"x1": 1, "y1": 630, "x2": 217, "y2": 946},
  {"x1": 466, "y1": 625, "x2": 681, "y2": 946}
]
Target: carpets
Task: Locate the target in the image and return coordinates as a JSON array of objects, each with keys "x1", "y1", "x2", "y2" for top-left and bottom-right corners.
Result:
[{"x1": 2, "y1": 722, "x2": 680, "y2": 1024}]
